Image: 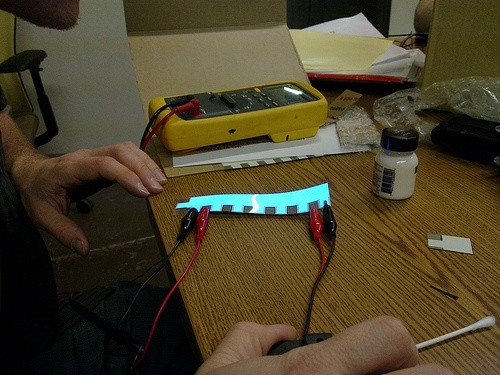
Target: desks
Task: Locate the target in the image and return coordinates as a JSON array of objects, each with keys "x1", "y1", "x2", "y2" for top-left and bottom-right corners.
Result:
[{"x1": 143, "y1": 89, "x2": 500, "y2": 375}]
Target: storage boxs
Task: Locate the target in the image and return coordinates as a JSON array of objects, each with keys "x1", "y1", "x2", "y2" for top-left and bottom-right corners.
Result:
[{"x1": 423, "y1": 0, "x2": 500, "y2": 85}]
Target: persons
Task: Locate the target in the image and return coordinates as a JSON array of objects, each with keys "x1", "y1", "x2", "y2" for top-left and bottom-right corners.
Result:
[{"x1": 0, "y1": 107, "x2": 456, "y2": 374}]
